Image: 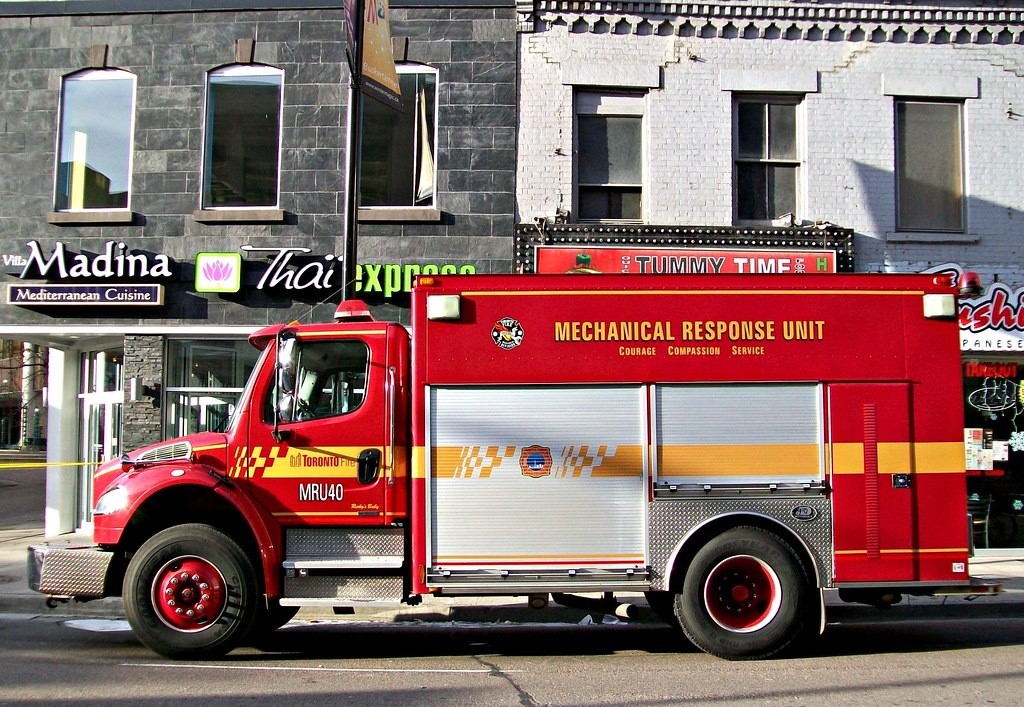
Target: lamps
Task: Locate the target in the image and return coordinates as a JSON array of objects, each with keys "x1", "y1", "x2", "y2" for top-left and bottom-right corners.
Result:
[{"x1": 129, "y1": 377, "x2": 144, "y2": 402}]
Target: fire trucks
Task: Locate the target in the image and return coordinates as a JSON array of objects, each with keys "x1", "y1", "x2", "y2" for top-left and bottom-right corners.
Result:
[{"x1": 26, "y1": 270, "x2": 1005, "y2": 660}]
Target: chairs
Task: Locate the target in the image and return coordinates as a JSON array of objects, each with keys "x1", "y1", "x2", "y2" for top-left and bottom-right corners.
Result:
[{"x1": 973, "y1": 493, "x2": 992, "y2": 548}]
